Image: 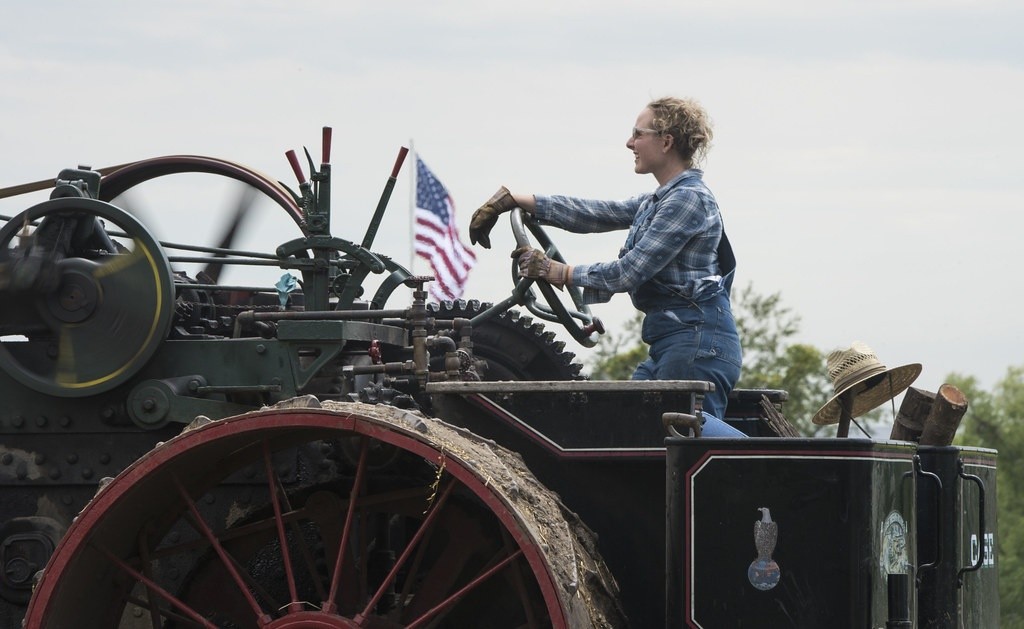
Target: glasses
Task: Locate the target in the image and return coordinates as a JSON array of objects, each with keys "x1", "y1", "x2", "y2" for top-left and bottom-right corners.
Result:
[{"x1": 633, "y1": 126, "x2": 662, "y2": 136}]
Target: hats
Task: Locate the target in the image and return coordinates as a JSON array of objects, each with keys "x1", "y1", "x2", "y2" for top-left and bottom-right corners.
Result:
[{"x1": 809, "y1": 340, "x2": 922, "y2": 425}]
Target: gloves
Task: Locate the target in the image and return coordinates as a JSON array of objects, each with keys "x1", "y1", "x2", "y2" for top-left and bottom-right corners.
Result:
[
  {"x1": 511, "y1": 247, "x2": 565, "y2": 292},
  {"x1": 469, "y1": 186, "x2": 519, "y2": 249}
]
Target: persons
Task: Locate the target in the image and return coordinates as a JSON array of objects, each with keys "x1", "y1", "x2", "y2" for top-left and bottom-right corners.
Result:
[{"x1": 468, "y1": 99, "x2": 743, "y2": 416}]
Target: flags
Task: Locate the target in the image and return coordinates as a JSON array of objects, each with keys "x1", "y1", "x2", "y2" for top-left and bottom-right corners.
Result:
[{"x1": 415, "y1": 156, "x2": 476, "y2": 307}]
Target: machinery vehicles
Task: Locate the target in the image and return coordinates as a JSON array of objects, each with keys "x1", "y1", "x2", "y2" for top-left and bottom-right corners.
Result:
[{"x1": 0, "y1": 125, "x2": 1001, "y2": 629}]
